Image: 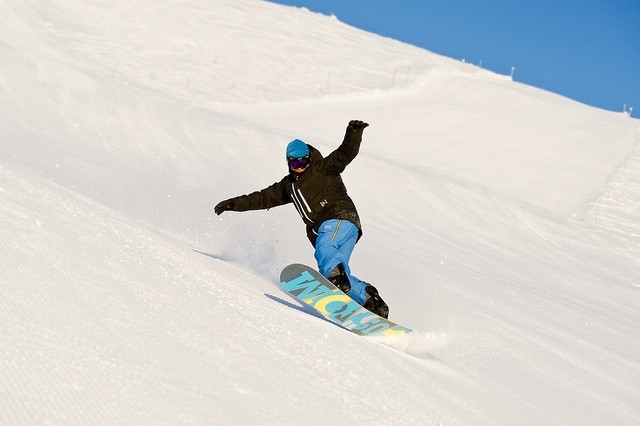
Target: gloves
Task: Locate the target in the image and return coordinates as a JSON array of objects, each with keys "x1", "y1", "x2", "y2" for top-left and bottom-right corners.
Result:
[
  {"x1": 214, "y1": 199, "x2": 234, "y2": 214},
  {"x1": 347, "y1": 120, "x2": 368, "y2": 131}
]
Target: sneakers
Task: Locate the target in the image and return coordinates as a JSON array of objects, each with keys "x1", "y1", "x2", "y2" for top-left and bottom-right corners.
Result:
[
  {"x1": 363, "y1": 282, "x2": 388, "y2": 319},
  {"x1": 328, "y1": 263, "x2": 351, "y2": 295}
]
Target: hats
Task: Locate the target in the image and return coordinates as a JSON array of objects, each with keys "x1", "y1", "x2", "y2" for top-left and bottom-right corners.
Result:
[{"x1": 286, "y1": 139, "x2": 309, "y2": 157}]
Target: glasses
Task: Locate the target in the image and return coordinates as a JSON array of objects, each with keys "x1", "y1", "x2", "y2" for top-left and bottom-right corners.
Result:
[{"x1": 288, "y1": 158, "x2": 308, "y2": 168}]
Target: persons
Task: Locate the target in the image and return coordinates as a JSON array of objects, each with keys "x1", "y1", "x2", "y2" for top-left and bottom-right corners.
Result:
[{"x1": 214, "y1": 120, "x2": 389, "y2": 320}]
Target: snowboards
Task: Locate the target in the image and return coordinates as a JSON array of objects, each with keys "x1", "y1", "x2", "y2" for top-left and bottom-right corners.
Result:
[{"x1": 279, "y1": 264, "x2": 412, "y2": 337}]
